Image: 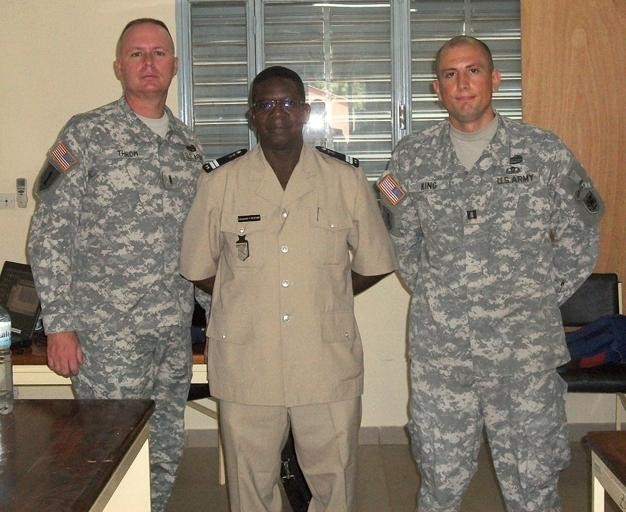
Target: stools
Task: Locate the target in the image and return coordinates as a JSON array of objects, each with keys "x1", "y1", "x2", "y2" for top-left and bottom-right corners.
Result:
[{"x1": 581, "y1": 431, "x2": 626, "y2": 512}]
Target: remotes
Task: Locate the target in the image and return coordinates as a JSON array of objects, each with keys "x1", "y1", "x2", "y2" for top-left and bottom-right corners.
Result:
[{"x1": 16, "y1": 178, "x2": 28, "y2": 208}]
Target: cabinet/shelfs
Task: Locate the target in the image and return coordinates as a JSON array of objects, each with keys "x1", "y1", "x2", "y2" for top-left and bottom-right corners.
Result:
[{"x1": 0, "y1": 398, "x2": 162, "y2": 512}]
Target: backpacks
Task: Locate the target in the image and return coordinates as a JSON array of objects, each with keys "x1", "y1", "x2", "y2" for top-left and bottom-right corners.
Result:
[{"x1": 554, "y1": 313, "x2": 626, "y2": 376}]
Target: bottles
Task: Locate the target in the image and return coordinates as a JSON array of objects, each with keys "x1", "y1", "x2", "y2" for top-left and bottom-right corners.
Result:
[{"x1": 0, "y1": 305, "x2": 14, "y2": 416}]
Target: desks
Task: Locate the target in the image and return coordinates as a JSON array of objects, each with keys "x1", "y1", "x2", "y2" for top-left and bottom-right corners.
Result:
[{"x1": 0, "y1": 351, "x2": 226, "y2": 486}]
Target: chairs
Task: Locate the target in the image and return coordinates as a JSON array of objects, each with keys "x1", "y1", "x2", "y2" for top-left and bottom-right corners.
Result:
[{"x1": 549, "y1": 272, "x2": 626, "y2": 431}]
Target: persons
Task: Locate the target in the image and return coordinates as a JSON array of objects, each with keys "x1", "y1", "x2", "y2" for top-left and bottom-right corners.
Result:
[
  {"x1": 174, "y1": 63, "x2": 401, "y2": 512},
  {"x1": 24, "y1": 16, "x2": 210, "y2": 512},
  {"x1": 372, "y1": 34, "x2": 606, "y2": 512}
]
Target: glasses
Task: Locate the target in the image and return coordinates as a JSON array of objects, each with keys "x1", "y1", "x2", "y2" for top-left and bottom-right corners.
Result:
[{"x1": 249, "y1": 98, "x2": 306, "y2": 113}]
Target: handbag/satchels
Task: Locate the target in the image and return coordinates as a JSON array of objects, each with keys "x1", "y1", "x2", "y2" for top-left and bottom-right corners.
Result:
[{"x1": 279, "y1": 435, "x2": 312, "y2": 512}]
